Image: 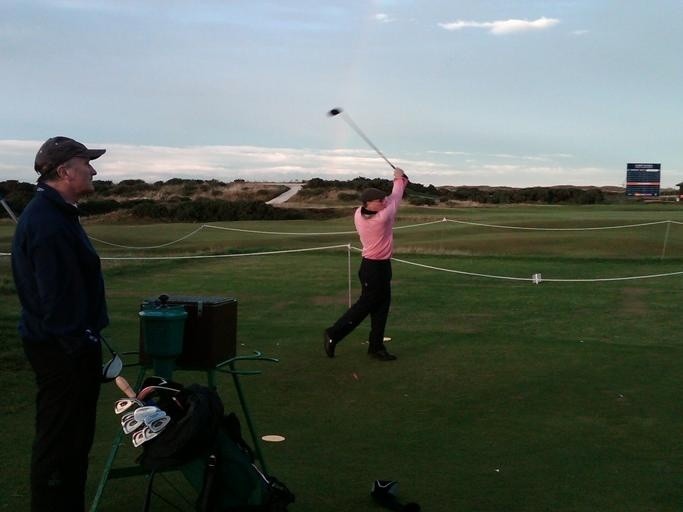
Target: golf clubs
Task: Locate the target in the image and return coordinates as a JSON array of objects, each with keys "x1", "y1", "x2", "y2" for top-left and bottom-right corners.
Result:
[
  {"x1": 330, "y1": 108, "x2": 409, "y2": 180},
  {"x1": 0, "y1": 200, "x2": 124, "y2": 380},
  {"x1": 113, "y1": 375, "x2": 171, "y2": 447}
]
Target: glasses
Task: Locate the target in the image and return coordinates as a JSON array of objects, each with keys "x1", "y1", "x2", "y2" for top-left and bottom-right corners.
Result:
[{"x1": 372, "y1": 199, "x2": 383, "y2": 202}]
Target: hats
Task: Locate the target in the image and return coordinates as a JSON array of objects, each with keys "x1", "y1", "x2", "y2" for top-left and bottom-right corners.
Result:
[
  {"x1": 361, "y1": 188, "x2": 386, "y2": 200},
  {"x1": 35, "y1": 136, "x2": 106, "y2": 176}
]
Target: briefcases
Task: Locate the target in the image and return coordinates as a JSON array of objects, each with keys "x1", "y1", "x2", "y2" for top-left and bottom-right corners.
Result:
[{"x1": 140, "y1": 295, "x2": 237, "y2": 363}]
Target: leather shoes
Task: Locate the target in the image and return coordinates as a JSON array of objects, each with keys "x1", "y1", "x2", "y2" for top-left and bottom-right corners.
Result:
[
  {"x1": 368, "y1": 351, "x2": 397, "y2": 361},
  {"x1": 324, "y1": 329, "x2": 335, "y2": 358}
]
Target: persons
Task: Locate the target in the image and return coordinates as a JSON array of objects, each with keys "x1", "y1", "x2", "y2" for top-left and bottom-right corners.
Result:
[
  {"x1": 11, "y1": 136, "x2": 109, "y2": 512},
  {"x1": 323, "y1": 167, "x2": 409, "y2": 361}
]
